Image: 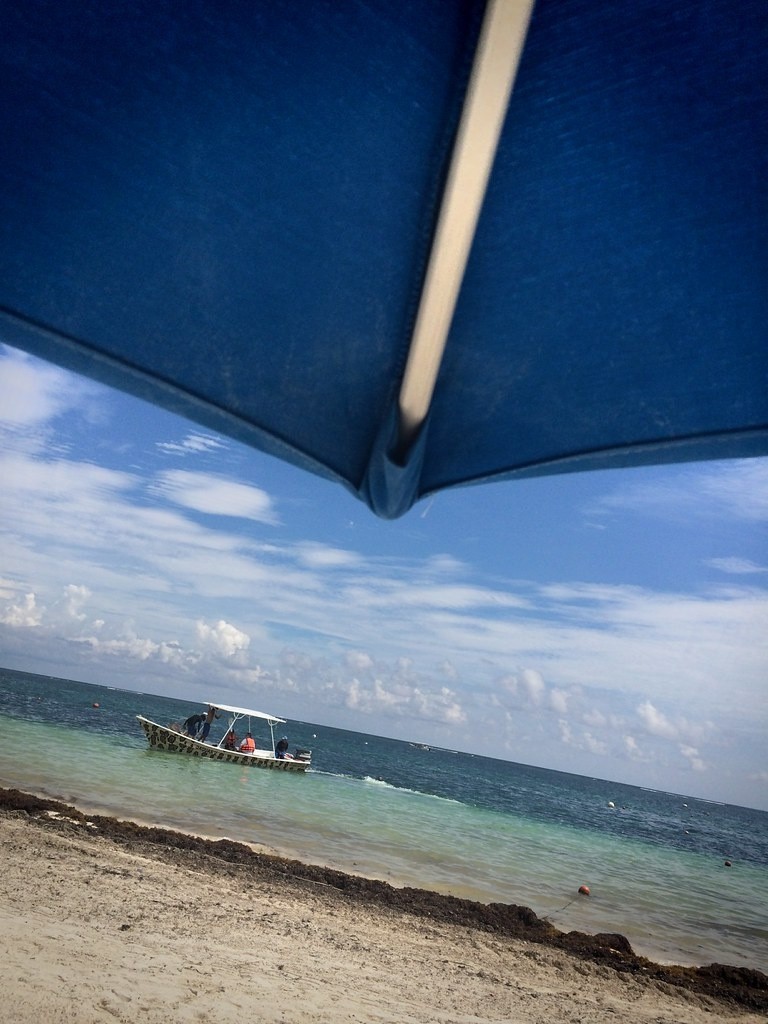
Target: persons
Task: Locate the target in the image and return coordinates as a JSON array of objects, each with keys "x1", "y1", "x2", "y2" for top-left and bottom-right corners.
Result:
[
  {"x1": 275, "y1": 737, "x2": 288, "y2": 758},
  {"x1": 198, "y1": 704, "x2": 222, "y2": 743},
  {"x1": 238, "y1": 733, "x2": 255, "y2": 755},
  {"x1": 225, "y1": 730, "x2": 237, "y2": 751},
  {"x1": 182, "y1": 712, "x2": 208, "y2": 739}
]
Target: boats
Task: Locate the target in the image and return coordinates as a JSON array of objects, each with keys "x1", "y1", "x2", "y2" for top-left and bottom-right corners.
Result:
[{"x1": 134, "y1": 701, "x2": 311, "y2": 773}]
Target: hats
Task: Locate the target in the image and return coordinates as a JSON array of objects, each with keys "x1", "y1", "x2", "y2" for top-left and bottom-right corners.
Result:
[
  {"x1": 201, "y1": 714, "x2": 206, "y2": 721},
  {"x1": 282, "y1": 736, "x2": 287, "y2": 739},
  {"x1": 245, "y1": 732, "x2": 253, "y2": 736}
]
